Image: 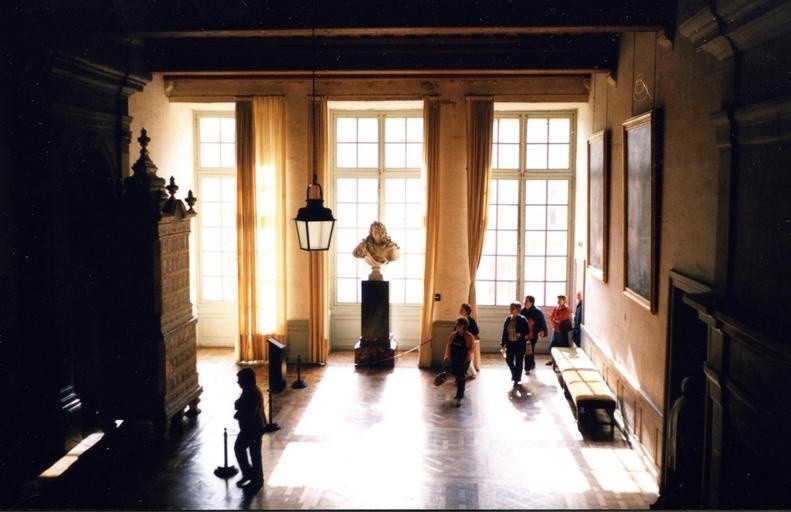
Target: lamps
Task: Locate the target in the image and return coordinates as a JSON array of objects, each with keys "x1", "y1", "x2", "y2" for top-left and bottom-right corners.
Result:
[{"x1": 292, "y1": 0, "x2": 338, "y2": 253}]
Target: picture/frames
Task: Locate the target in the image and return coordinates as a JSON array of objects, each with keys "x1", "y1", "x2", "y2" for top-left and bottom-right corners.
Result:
[
  {"x1": 588, "y1": 127, "x2": 609, "y2": 285},
  {"x1": 622, "y1": 110, "x2": 664, "y2": 314}
]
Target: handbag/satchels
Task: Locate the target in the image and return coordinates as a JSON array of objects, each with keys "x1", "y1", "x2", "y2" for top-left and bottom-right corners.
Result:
[
  {"x1": 433, "y1": 372, "x2": 447, "y2": 386},
  {"x1": 560, "y1": 320, "x2": 572, "y2": 332},
  {"x1": 239, "y1": 418, "x2": 265, "y2": 432}
]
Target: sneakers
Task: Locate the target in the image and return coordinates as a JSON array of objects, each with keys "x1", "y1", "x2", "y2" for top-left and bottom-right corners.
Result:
[
  {"x1": 455, "y1": 399, "x2": 463, "y2": 407},
  {"x1": 237, "y1": 471, "x2": 255, "y2": 484},
  {"x1": 546, "y1": 360, "x2": 553, "y2": 365},
  {"x1": 525, "y1": 341, "x2": 533, "y2": 355}
]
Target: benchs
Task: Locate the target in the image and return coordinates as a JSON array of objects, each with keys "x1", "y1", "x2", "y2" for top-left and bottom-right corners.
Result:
[{"x1": 547, "y1": 342, "x2": 617, "y2": 441}]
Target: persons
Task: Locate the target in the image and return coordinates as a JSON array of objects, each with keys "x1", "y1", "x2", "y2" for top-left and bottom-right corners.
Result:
[
  {"x1": 351, "y1": 222, "x2": 398, "y2": 264},
  {"x1": 544, "y1": 295, "x2": 570, "y2": 365},
  {"x1": 451, "y1": 302, "x2": 478, "y2": 383},
  {"x1": 232, "y1": 368, "x2": 267, "y2": 488},
  {"x1": 442, "y1": 317, "x2": 477, "y2": 408},
  {"x1": 647, "y1": 376, "x2": 704, "y2": 511},
  {"x1": 572, "y1": 291, "x2": 582, "y2": 347},
  {"x1": 519, "y1": 295, "x2": 548, "y2": 375},
  {"x1": 499, "y1": 302, "x2": 531, "y2": 388}
]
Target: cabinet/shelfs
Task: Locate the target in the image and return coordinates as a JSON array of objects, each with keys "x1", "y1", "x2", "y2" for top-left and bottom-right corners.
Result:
[{"x1": 350, "y1": 279, "x2": 398, "y2": 370}]
